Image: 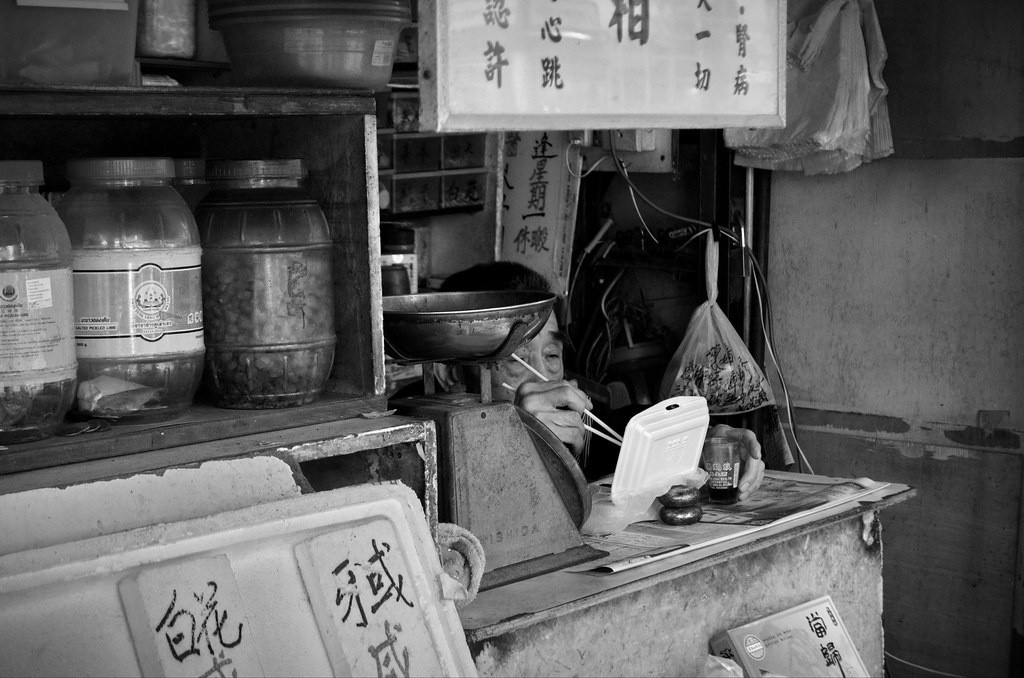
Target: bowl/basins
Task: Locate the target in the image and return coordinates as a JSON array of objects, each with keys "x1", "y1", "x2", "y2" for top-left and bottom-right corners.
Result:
[
  {"x1": 383, "y1": 290, "x2": 557, "y2": 361},
  {"x1": 207, "y1": 0, "x2": 413, "y2": 90}
]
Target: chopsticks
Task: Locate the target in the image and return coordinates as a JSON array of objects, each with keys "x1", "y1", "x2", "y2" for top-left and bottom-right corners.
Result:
[{"x1": 502, "y1": 351, "x2": 624, "y2": 447}]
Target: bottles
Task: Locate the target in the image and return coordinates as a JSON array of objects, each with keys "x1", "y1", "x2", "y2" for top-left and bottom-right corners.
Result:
[
  {"x1": 0, "y1": 160, "x2": 79, "y2": 447},
  {"x1": 138, "y1": 0, "x2": 195, "y2": 55},
  {"x1": 54, "y1": 157, "x2": 206, "y2": 426},
  {"x1": 194, "y1": 158, "x2": 338, "y2": 410}
]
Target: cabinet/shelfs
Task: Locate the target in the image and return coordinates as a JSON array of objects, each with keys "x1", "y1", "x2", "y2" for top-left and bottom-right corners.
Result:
[{"x1": 0, "y1": 73, "x2": 489, "y2": 549}]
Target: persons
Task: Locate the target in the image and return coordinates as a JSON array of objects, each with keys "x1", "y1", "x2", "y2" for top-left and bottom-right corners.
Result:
[{"x1": 388, "y1": 259, "x2": 764, "y2": 503}]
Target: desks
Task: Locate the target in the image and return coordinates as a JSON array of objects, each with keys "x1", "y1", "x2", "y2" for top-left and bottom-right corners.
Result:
[{"x1": 457, "y1": 472, "x2": 917, "y2": 678}]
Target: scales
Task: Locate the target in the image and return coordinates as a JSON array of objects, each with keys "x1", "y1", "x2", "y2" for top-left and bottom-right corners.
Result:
[{"x1": 383, "y1": 290, "x2": 612, "y2": 593}]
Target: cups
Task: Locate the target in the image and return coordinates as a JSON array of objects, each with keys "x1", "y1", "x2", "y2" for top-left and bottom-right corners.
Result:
[{"x1": 702, "y1": 437, "x2": 742, "y2": 505}]
